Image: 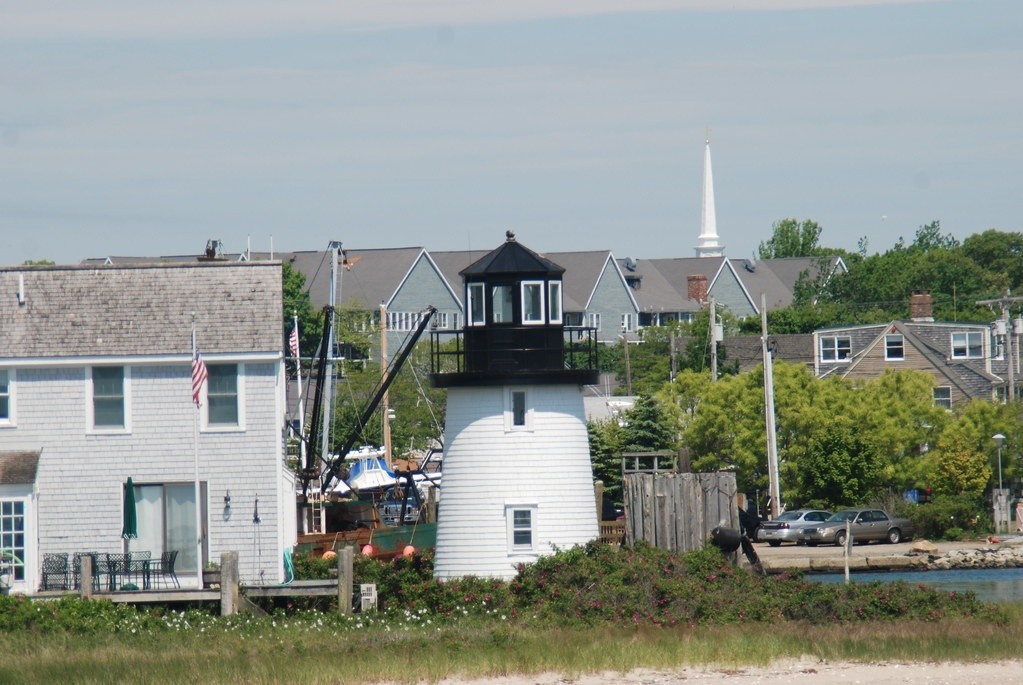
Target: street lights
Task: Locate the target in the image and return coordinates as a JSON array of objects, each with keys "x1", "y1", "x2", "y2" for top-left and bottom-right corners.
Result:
[{"x1": 992, "y1": 433, "x2": 1007, "y2": 535}]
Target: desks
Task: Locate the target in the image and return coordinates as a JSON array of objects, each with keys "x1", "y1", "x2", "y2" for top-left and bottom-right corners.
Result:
[{"x1": 94, "y1": 558, "x2": 162, "y2": 592}]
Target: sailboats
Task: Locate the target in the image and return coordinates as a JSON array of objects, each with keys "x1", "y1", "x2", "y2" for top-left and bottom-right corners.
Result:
[{"x1": 288, "y1": 240, "x2": 444, "y2": 532}]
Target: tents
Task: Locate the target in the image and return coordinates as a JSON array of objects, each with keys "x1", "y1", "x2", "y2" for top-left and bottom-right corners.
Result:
[{"x1": 333, "y1": 459, "x2": 407, "y2": 494}]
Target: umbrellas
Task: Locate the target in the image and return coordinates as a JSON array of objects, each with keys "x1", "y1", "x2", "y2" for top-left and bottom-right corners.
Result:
[{"x1": 121, "y1": 476, "x2": 139, "y2": 540}]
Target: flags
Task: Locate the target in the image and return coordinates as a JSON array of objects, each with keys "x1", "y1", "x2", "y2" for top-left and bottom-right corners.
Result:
[
  {"x1": 289, "y1": 326, "x2": 296, "y2": 357},
  {"x1": 190, "y1": 329, "x2": 209, "y2": 409}
]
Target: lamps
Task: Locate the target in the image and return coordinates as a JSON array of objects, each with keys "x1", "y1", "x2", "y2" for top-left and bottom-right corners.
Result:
[{"x1": 224, "y1": 490, "x2": 230, "y2": 508}]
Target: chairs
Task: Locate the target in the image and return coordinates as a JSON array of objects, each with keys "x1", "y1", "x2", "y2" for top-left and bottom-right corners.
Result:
[{"x1": 41, "y1": 550, "x2": 181, "y2": 592}]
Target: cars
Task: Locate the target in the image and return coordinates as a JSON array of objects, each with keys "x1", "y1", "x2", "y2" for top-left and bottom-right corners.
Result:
[
  {"x1": 757, "y1": 509, "x2": 841, "y2": 547},
  {"x1": 803, "y1": 509, "x2": 914, "y2": 546},
  {"x1": 378, "y1": 487, "x2": 426, "y2": 525}
]
